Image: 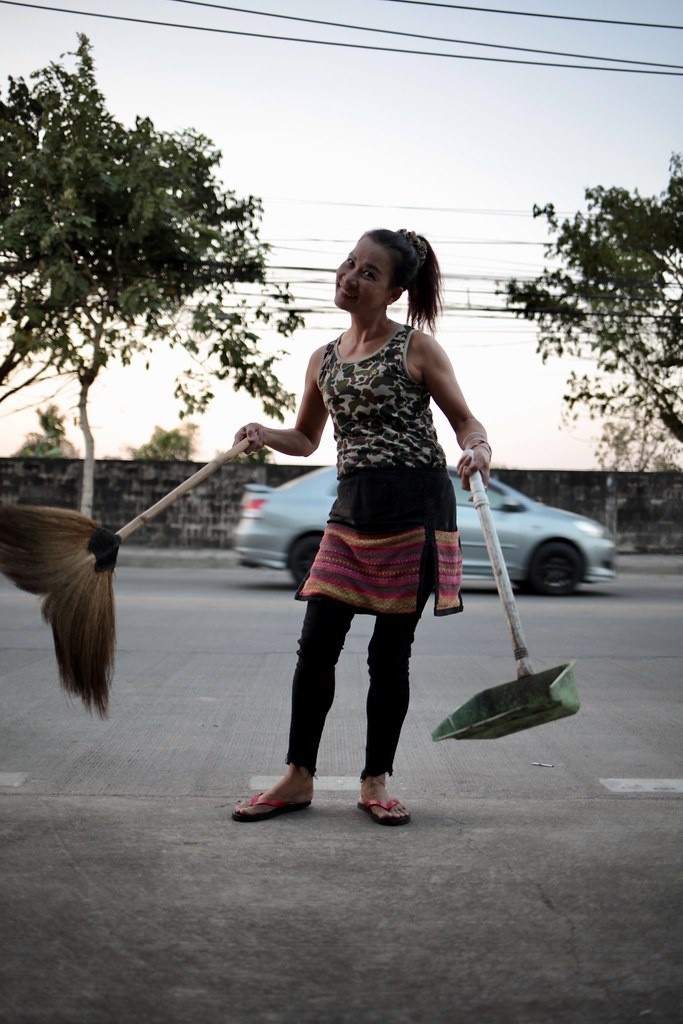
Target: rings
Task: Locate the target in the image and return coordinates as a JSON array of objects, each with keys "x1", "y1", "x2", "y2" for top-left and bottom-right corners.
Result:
[{"x1": 484, "y1": 486, "x2": 488, "y2": 493}]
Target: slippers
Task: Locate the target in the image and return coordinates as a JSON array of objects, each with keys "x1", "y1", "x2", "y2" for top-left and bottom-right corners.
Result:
[
  {"x1": 232, "y1": 793, "x2": 311, "y2": 822},
  {"x1": 357, "y1": 798, "x2": 410, "y2": 826}
]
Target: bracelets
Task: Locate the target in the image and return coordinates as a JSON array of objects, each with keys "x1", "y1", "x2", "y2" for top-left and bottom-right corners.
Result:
[{"x1": 460, "y1": 431, "x2": 493, "y2": 459}]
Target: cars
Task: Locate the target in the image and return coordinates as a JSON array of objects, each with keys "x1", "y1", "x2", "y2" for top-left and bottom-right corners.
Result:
[{"x1": 232, "y1": 461, "x2": 616, "y2": 594}]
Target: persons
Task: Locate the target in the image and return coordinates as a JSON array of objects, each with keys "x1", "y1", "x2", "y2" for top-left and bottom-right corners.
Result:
[{"x1": 230, "y1": 228, "x2": 493, "y2": 824}]
